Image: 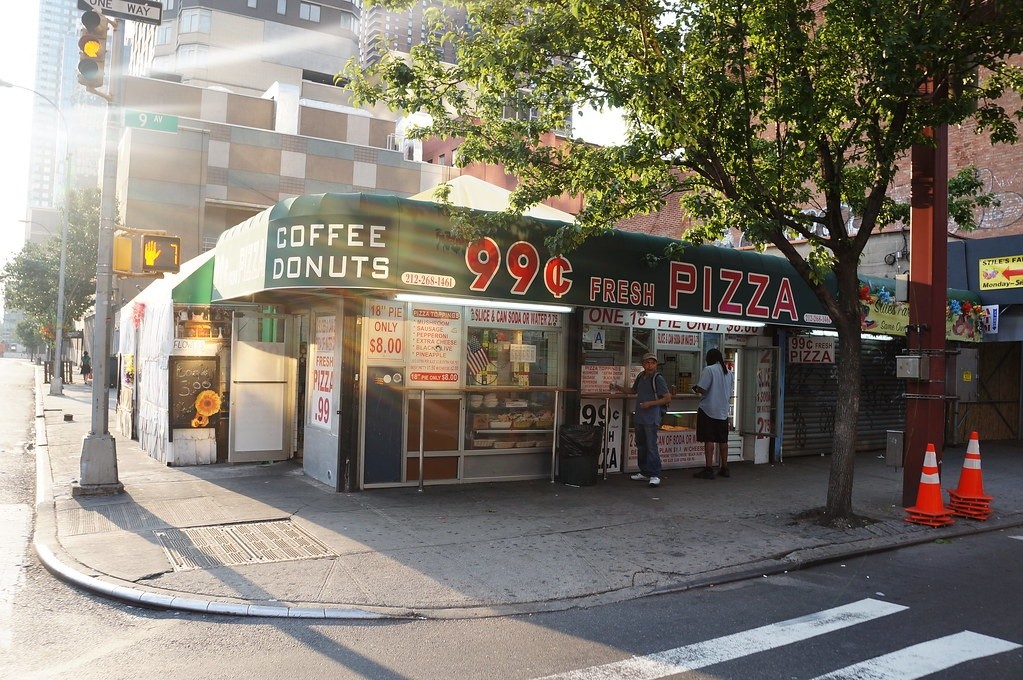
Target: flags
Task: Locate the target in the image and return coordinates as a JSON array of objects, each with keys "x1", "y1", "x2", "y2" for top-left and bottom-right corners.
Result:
[{"x1": 466, "y1": 334, "x2": 489, "y2": 376}]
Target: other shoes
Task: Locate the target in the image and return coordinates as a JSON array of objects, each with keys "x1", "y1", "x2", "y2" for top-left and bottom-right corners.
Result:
[
  {"x1": 694, "y1": 467, "x2": 714, "y2": 480},
  {"x1": 719, "y1": 467, "x2": 730, "y2": 477},
  {"x1": 85, "y1": 381, "x2": 87, "y2": 384}
]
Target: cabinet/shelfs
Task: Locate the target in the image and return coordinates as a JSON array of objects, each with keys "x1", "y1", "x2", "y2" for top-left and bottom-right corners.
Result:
[{"x1": 463, "y1": 390, "x2": 558, "y2": 484}]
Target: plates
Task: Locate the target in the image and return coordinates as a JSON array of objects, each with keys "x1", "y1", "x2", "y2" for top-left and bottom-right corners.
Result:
[{"x1": 469, "y1": 393, "x2": 498, "y2": 408}]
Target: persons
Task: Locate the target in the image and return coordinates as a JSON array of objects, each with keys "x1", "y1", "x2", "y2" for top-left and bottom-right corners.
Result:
[
  {"x1": 616, "y1": 353, "x2": 671, "y2": 486},
  {"x1": 692, "y1": 348, "x2": 734, "y2": 480},
  {"x1": 80, "y1": 350, "x2": 91, "y2": 384}
]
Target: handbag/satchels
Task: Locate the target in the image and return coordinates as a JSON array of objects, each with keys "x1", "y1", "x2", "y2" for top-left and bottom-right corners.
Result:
[{"x1": 89, "y1": 369, "x2": 93, "y2": 379}]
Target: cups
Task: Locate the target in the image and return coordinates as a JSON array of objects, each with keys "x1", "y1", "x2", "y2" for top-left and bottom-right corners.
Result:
[
  {"x1": 672, "y1": 386, "x2": 677, "y2": 395},
  {"x1": 610, "y1": 381, "x2": 617, "y2": 395}
]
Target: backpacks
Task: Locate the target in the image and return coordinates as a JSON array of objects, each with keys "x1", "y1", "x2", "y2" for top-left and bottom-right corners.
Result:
[{"x1": 653, "y1": 372, "x2": 672, "y2": 416}]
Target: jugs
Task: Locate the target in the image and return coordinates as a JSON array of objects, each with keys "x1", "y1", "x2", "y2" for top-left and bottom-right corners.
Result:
[{"x1": 178, "y1": 310, "x2": 189, "y2": 320}]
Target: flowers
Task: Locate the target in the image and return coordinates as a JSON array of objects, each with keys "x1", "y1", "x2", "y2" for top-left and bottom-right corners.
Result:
[
  {"x1": 859, "y1": 279, "x2": 988, "y2": 334},
  {"x1": 190, "y1": 387, "x2": 222, "y2": 416},
  {"x1": 124, "y1": 356, "x2": 136, "y2": 384},
  {"x1": 130, "y1": 301, "x2": 147, "y2": 332}
]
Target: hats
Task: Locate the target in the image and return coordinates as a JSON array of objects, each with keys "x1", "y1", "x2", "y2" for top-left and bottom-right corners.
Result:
[
  {"x1": 642, "y1": 352, "x2": 657, "y2": 363},
  {"x1": 83, "y1": 351, "x2": 89, "y2": 354}
]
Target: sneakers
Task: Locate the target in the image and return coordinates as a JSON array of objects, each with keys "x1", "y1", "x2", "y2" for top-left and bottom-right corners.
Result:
[
  {"x1": 649, "y1": 477, "x2": 660, "y2": 487},
  {"x1": 631, "y1": 473, "x2": 650, "y2": 481}
]
